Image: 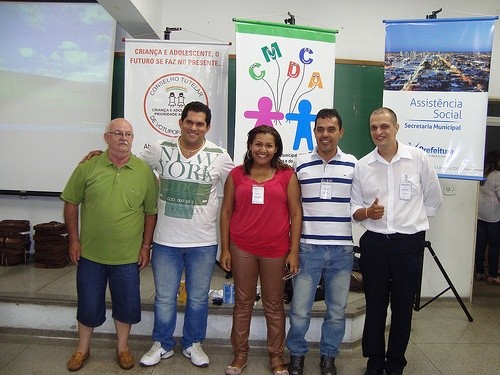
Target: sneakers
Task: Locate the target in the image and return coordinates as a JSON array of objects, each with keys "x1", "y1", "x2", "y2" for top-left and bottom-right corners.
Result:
[
  {"x1": 289, "y1": 354, "x2": 305, "y2": 375},
  {"x1": 139, "y1": 341, "x2": 174, "y2": 366},
  {"x1": 319, "y1": 355, "x2": 337, "y2": 375},
  {"x1": 183, "y1": 341, "x2": 210, "y2": 368}
]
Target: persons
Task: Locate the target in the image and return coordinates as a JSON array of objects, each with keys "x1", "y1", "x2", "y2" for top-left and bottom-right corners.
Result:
[
  {"x1": 292, "y1": 108, "x2": 358, "y2": 375},
  {"x1": 61, "y1": 118, "x2": 159, "y2": 371},
  {"x1": 79, "y1": 101, "x2": 235, "y2": 368},
  {"x1": 220, "y1": 125, "x2": 302, "y2": 375},
  {"x1": 350, "y1": 108, "x2": 442, "y2": 375},
  {"x1": 475, "y1": 151, "x2": 500, "y2": 286}
]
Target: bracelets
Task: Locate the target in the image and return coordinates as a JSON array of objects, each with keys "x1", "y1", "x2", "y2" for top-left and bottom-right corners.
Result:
[{"x1": 366, "y1": 208, "x2": 368, "y2": 217}]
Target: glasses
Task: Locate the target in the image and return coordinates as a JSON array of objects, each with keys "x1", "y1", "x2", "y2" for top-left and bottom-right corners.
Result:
[{"x1": 106, "y1": 131, "x2": 133, "y2": 138}]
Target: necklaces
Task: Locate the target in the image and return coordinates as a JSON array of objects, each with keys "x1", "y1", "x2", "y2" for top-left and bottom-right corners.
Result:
[{"x1": 179, "y1": 140, "x2": 204, "y2": 155}]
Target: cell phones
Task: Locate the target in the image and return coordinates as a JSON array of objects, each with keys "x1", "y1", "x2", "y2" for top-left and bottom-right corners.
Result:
[{"x1": 286, "y1": 264, "x2": 291, "y2": 272}]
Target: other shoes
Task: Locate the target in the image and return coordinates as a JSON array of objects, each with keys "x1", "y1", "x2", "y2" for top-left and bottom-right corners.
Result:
[
  {"x1": 364, "y1": 365, "x2": 404, "y2": 375},
  {"x1": 115, "y1": 346, "x2": 134, "y2": 370},
  {"x1": 226, "y1": 361, "x2": 247, "y2": 375},
  {"x1": 67, "y1": 347, "x2": 91, "y2": 371},
  {"x1": 269, "y1": 365, "x2": 289, "y2": 375}
]
